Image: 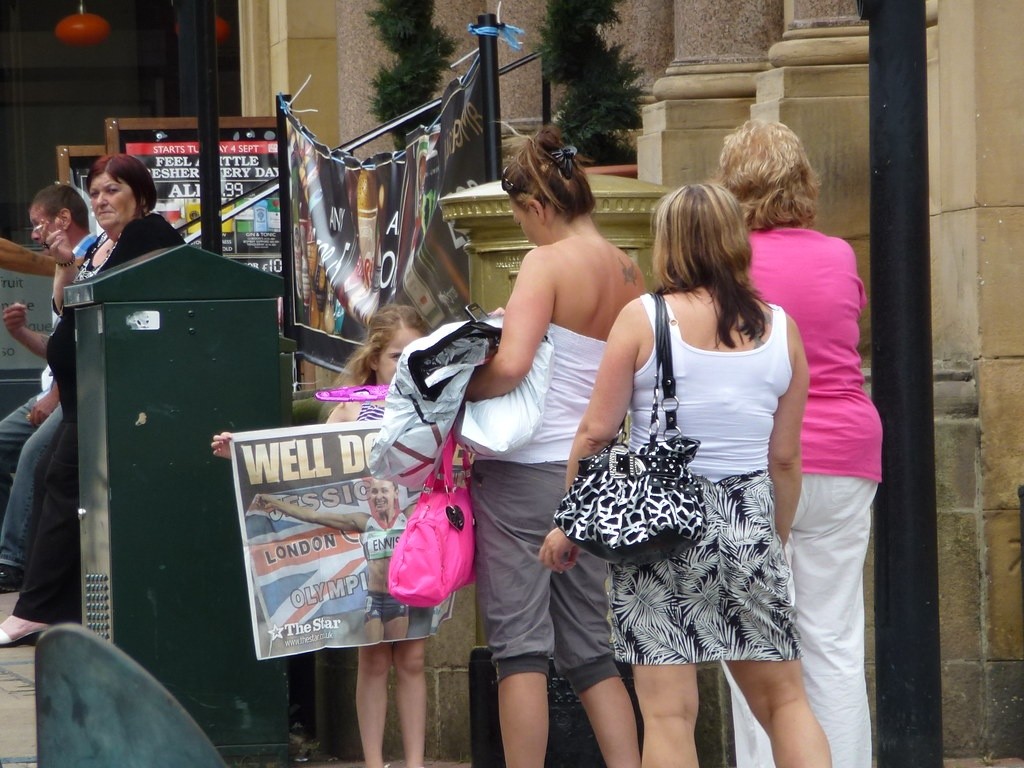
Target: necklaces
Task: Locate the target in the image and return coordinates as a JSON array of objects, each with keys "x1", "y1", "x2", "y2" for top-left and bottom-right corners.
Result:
[{"x1": 107, "y1": 241, "x2": 118, "y2": 253}]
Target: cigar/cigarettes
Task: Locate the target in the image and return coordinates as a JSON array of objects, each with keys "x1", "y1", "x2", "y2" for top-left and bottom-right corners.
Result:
[{"x1": 33, "y1": 221, "x2": 48, "y2": 232}]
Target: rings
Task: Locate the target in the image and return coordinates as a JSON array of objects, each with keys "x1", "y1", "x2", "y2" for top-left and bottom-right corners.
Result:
[{"x1": 42, "y1": 241, "x2": 49, "y2": 250}]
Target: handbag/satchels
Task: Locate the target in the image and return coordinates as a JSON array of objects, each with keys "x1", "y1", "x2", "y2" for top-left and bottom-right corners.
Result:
[
  {"x1": 384, "y1": 432, "x2": 479, "y2": 606},
  {"x1": 551, "y1": 435, "x2": 706, "y2": 562}
]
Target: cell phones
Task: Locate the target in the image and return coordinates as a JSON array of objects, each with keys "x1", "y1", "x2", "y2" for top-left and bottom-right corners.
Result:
[{"x1": 465, "y1": 302, "x2": 488, "y2": 322}]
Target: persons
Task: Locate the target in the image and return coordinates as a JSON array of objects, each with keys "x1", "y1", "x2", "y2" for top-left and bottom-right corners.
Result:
[
  {"x1": 1, "y1": 185, "x2": 102, "y2": 592},
  {"x1": 210, "y1": 306, "x2": 435, "y2": 767},
  {"x1": 260, "y1": 476, "x2": 420, "y2": 643},
  {"x1": 1, "y1": 153, "x2": 188, "y2": 651},
  {"x1": 451, "y1": 124, "x2": 641, "y2": 768},
  {"x1": 539, "y1": 184, "x2": 835, "y2": 766},
  {"x1": 707, "y1": 117, "x2": 883, "y2": 767}
]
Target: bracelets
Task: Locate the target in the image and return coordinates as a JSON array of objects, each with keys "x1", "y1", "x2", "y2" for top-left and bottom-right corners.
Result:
[{"x1": 55, "y1": 250, "x2": 76, "y2": 267}]
